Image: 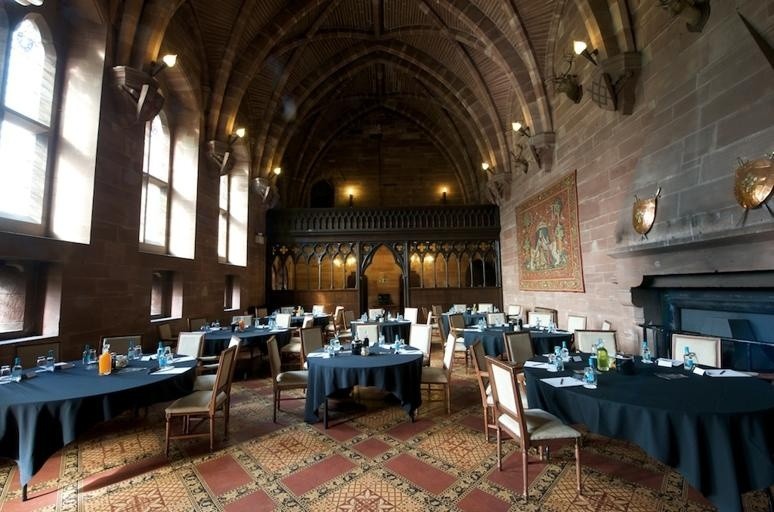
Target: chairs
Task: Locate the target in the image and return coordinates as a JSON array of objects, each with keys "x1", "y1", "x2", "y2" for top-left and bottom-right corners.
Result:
[
  {"x1": 97, "y1": 300, "x2": 617, "y2": 390},
  {"x1": 670, "y1": 334, "x2": 723, "y2": 370},
  {"x1": 470, "y1": 338, "x2": 494, "y2": 444},
  {"x1": 484, "y1": 354, "x2": 582, "y2": 502},
  {"x1": 164, "y1": 345, "x2": 237, "y2": 455},
  {"x1": 16, "y1": 342, "x2": 60, "y2": 367},
  {"x1": 416, "y1": 331, "x2": 457, "y2": 417},
  {"x1": 266, "y1": 334, "x2": 310, "y2": 424}
]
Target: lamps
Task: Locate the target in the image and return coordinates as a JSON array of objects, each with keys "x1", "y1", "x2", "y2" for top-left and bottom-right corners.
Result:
[
  {"x1": 572, "y1": 40, "x2": 598, "y2": 67},
  {"x1": 441, "y1": 187, "x2": 449, "y2": 201},
  {"x1": 511, "y1": 122, "x2": 530, "y2": 138},
  {"x1": 151, "y1": 54, "x2": 179, "y2": 77},
  {"x1": 228, "y1": 128, "x2": 246, "y2": 145},
  {"x1": 482, "y1": 163, "x2": 495, "y2": 176},
  {"x1": 349, "y1": 189, "x2": 353, "y2": 208},
  {"x1": 269, "y1": 167, "x2": 282, "y2": 180}
]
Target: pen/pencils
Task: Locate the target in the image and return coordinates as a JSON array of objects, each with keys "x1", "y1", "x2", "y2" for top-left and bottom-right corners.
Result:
[
  {"x1": 534, "y1": 364, "x2": 544, "y2": 366},
  {"x1": 561, "y1": 379, "x2": 564, "y2": 384},
  {"x1": 720, "y1": 371, "x2": 725, "y2": 374}
]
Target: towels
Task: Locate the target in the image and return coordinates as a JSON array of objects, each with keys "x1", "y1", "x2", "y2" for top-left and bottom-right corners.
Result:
[
  {"x1": 705, "y1": 369, "x2": 759, "y2": 378},
  {"x1": 39, "y1": 361, "x2": 67, "y2": 372}
]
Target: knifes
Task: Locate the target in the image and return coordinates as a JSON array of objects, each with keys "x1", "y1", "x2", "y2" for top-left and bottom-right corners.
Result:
[{"x1": 147, "y1": 368, "x2": 161, "y2": 375}]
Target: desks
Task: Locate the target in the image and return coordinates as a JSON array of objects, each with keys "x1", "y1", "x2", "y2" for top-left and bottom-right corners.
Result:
[{"x1": 307, "y1": 342, "x2": 424, "y2": 430}]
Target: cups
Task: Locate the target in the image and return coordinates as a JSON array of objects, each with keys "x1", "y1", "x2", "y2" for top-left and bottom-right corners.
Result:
[
  {"x1": 0, "y1": 366, "x2": 11, "y2": 385},
  {"x1": 164, "y1": 347, "x2": 170, "y2": 359},
  {"x1": 158, "y1": 356, "x2": 167, "y2": 370},
  {"x1": 35, "y1": 357, "x2": 46, "y2": 373},
  {"x1": 201, "y1": 325, "x2": 210, "y2": 332},
  {"x1": 89, "y1": 349, "x2": 96, "y2": 364},
  {"x1": 134, "y1": 345, "x2": 143, "y2": 358}
]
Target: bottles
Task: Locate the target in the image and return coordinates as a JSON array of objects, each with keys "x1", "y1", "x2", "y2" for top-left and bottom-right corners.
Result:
[
  {"x1": 682, "y1": 346, "x2": 693, "y2": 370},
  {"x1": 11, "y1": 357, "x2": 23, "y2": 383},
  {"x1": 327, "y1": 333, "x2": 405, "y2": 358},
  {"x1": 46, "y1": 350, "x2": 55, "y2": 372},
  {"x1": 477, "y1": 316, "x2": 556, "y2": 333},
  {"x1": 292, "y1": 305, "x2": 305, "y2": 317},
  {"x1": 98, "y1": 345, "x2": 113, "y2": 376},
  {"x1": 361, "y1": 310, "x2": 403, "y2": 324},
  {"x1": 466, "y1": 303, "x2": 477, "y2": 315},
  {"x1": 156, "y1": 340, "x2": 163, "y2": 360},
  {"x1": 230, "y1": 319, "x2": 275, "y2": 333},
  {"x1": 215, "y1": 320, "x2": 219, "y2": 328},
  {"x1": 82, "y1": 344, "x2": 90, "y2": 364},
  {"x1": 128, "y1": 340, "x2": 135, "y2": 360},
  {"x1": 547, "y1": 338, "x2": 651, "y2": 390}
]
